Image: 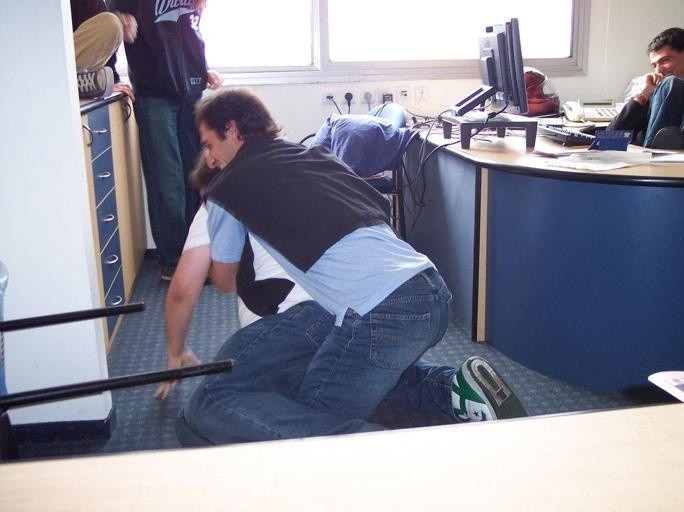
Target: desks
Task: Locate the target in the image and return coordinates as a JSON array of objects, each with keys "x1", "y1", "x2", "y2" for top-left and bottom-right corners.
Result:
[
  {"x1": 0, "y1": 402, "x2": 684, "y2": 512},
  {"x1": 395, "y1": 114, "x2": 683, "y2": 401}
]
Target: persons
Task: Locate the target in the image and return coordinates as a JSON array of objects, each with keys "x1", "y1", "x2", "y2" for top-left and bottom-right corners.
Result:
[
  {"x1": 606, "y1": 28, "x2": 684, "y2": 149},
  {"x1": 105, "y1": 0, "x2": 222, "y2": 285},
  {"x1": 153, "y1": 150, "x2": 390, "y2": 445},
  {"x1": 198, "y1": 89, "x2": 528, "y2": 420},
  {"x1": 70, "y1": 0, "x2": 136, "y2": 105}
]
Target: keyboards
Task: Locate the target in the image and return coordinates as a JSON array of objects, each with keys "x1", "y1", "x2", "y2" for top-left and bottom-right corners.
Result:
[{"x1": 538, "y1": 124, "x2": 595, "y2": 148}]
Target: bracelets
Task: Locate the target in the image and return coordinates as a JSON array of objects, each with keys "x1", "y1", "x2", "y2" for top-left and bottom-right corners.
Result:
[{"x1": 633, "y1": 92, "x2": 646, "y2": 104}]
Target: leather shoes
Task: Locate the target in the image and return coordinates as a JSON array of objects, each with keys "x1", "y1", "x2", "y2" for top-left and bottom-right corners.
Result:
[{"x1": 651, "y1": 125, "x2": 683, "y2": 149}]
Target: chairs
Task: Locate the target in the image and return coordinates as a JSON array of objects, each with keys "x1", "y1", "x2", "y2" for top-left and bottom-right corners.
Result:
[{"x1": 358, "y1": 101, "x2": 408, "y2": 244}]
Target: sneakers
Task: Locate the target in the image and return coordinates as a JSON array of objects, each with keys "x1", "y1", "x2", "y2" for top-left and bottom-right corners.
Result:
[
  {"x1": 76, "y1": 66, "x2": 114, "y2": 99},
  {"x1": 450, "y1": 355, "x2": 528, "y2": 422},
  {"x1": 160, "y1": 265, "x2": 175, "y2": 280}
]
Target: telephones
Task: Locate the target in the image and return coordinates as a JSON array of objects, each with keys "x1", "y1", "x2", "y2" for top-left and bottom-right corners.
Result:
[{"x1": 562, "y1": 96, "x2": 620, "y2": 122}]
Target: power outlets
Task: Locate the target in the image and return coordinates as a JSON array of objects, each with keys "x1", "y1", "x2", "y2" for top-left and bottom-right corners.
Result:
[
  {"x1": 359, "y1": 87, "x2": 375, "y2": 104},
  {"x1": 323, "y1": 90, "x2": 336, "y2": 105},
  {"x1": 378, "y1": 88, "x2": 395, "y2": 106},
  {"x1": 338, "y1": 88, "x2": 356, "y2": 104}
]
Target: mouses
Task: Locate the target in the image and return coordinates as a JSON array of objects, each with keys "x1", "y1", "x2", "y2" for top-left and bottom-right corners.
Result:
[{"x1": 580, "y1": 125, "x2": 596, "y2": 135}]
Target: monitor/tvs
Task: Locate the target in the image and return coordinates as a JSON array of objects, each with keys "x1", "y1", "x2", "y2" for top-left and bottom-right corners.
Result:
[{"x1": 451, "y1": 17, "x2": 528, "y2": 114}]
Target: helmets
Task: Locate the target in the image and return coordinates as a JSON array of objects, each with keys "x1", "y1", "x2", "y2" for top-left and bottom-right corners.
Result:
[{"x1": 523, "y1": 66, "x2": 560, "y2": 117}]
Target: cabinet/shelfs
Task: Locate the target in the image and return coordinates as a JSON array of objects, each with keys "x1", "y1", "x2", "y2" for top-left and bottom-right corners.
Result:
[
  {"x1": 108, "y1": 94, "x2": 147, "y2": 305},
  {"x1": 80, "y1": 104, "x2": 127, "y2": 354}
]
